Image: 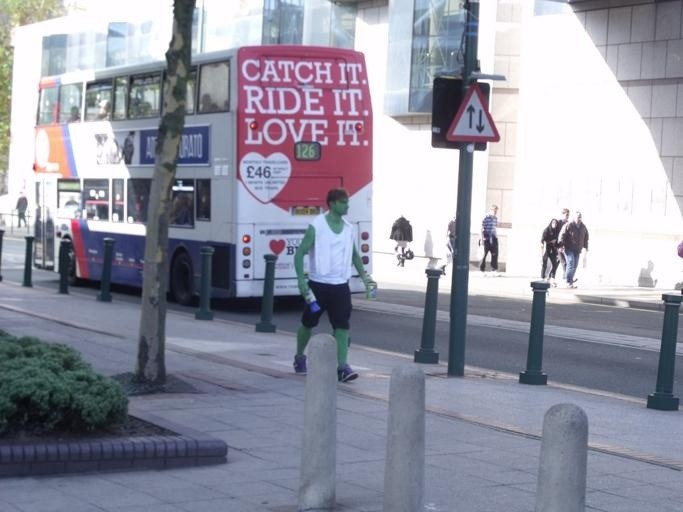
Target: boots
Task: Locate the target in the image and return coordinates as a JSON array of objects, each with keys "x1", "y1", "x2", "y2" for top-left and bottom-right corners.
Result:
[
  {"x1": 440, "y1": 265, "x2": 446, "y2": 275},
  {"x1": 396, "y1": 254, "x2": 406, "y2": 267}
]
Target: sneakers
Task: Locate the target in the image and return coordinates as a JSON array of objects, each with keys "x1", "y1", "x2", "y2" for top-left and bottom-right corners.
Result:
[
  {"x1": 337, "y1": 363, "x2": 358, "y2": 383},
  {"x1": 490, "y1": 270, "x2": 501, "y2": 277},
  {"x1": 479, "y1": 271, "x2": 488, "y2": 276},
  {"x1": 293, "y1": 353, "x2": 307, "y2": 375},
  {"x1": 561, "y1": 278, "x2": 577, "y2": 289},
  {"x1": 547, "y1": 277, "x2": 557, "y2": 288}
]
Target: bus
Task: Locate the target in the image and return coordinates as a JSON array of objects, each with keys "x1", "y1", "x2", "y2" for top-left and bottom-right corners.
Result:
[{"x1": 26, "y1": 41, "x2": 379, "y2": 311}]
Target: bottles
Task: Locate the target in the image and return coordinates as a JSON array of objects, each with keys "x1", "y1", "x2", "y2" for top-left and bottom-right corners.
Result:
[
  {"x1": 302, "y1": 288, "x2": 324, "y2": 312},
  {"x1": 365, "y1": 282, "x2": 380, "y2": 301}
]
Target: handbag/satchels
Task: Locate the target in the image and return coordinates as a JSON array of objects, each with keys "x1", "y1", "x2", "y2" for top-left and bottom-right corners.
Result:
[{"x1": 550, "y1": 239, "x2": 556, "y2": 247}]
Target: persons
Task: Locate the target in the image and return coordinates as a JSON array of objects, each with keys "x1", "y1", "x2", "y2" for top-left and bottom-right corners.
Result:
[
  {"x1": 440, "y1": 216, "x2": 455, "y2": 277},
  {"x1": 389, "y1": 210, "x2": 412, "y2": 267},
  {"x1": 121, "y1": 131, "x2": 135, "y2": 164},
  {"x1": 65, "y1": 93, "x2": 219, "y2": 122},
  {"x1": 547, "y1": 208, "x2": 571, "y2": 281},
  {"x1": 14, "y1": 192, "x2": 28, "y2": 229},
  {"x1": 538, "y1": 218, "x2": 560, "y2": 282},
  {"x1": 63, "y1": 179, "x2": 209, "y2": 227},
  {"x1": 86, "y1": 132, "x2": 114, "y2": 164},
  {"x1": 560, "y1": 210, "x2": 591, "y2": 288},
  {"x1": 292, "y1": 188, "x2": 376, "y2": 384},
  {"x1": 478, "y1": 205, "x2": 504, "y2": 276}
]
